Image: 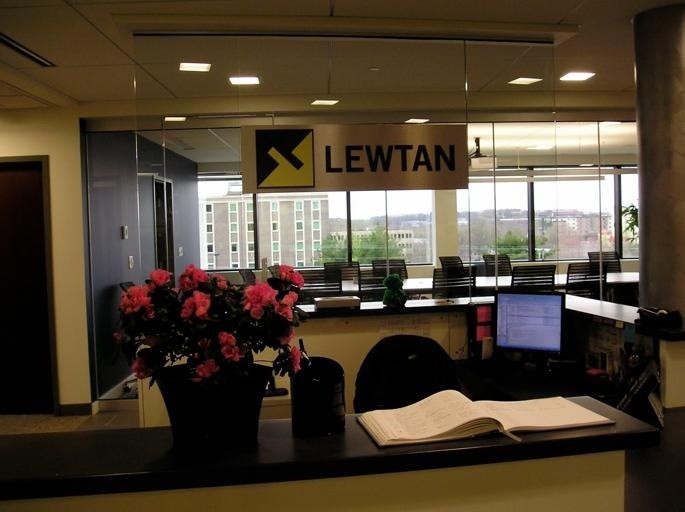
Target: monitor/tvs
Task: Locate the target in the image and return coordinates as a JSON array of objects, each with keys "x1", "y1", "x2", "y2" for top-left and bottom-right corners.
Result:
[{"x1": 493, "y1": 290, "x2": 565, "y2": 356}]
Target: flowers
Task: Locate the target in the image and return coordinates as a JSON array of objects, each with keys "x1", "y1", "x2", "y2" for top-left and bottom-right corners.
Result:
[{"x1": 116, "y1": 264, "x2": 305, "y2": 383}]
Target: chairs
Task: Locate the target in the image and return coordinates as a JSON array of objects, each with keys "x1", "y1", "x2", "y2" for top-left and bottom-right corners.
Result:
[
  {"x1": 323, "y1": 261, "x2": 361, "y2": 282},
  {"x1": 372, "y1": 259, "x2": 409, "y2": 279},
  {"x1": 510, "y1": 265, "x2": 557, "y2": 294},
  {"x1": 352, "y1": 334, "x2": 459, "y2": 415},
  {"x1": 297, "y1": 268, "x2": 343, "y2": 305},
  {"x1": 587, "y1": 251, "x2": 622, "y2": 272},
  {"x1": 239, "y1": 269, "x2": 257, "y2": 286},
  {"x1": 483, "y1": 253, "x2": 512, "y2": 276},
  {"x1": 439, "y1": 256, "x2": 466, "y2": 277},
  {"x1": 565, "y1": 261, "x2": 609, "y2": 301},
  {"x1": 431, "y1": 264, "x2": 478, "y2": 299},
  {"x1": 357, "y1": 267, "x2": 403, "y2": 302}
]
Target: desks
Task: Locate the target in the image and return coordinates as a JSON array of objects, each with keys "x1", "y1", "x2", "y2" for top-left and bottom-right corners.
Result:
[{"x1": 300, "y1": 270, "x2": 639, "y2": 307}]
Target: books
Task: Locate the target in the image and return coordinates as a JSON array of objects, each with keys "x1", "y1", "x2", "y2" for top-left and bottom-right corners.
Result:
[{"x1": 356, "y1": 389, "x2": 618, "y2": 447}]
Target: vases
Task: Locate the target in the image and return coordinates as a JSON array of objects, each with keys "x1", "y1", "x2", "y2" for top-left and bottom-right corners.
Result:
[{"x1": 157, "y1": 361, "x2": 273, "y2": 456}]
